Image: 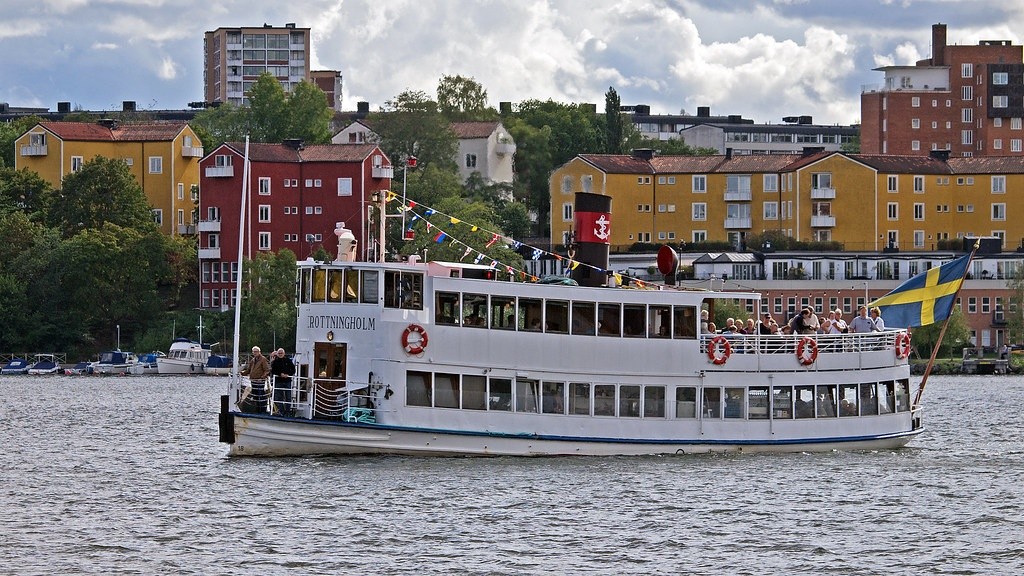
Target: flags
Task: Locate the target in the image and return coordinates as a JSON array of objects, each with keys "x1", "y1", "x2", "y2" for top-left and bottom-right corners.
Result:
[
  {"x1": 385, "y1": 191, "x2": 645, "y2": 291},
  {"x1": 866, "y1": 252, "x2": 973, "y2": 330}
]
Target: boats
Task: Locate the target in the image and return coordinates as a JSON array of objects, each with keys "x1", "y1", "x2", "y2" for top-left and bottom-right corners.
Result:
[
  {"x1": 1, "y1": 359, "x2": 33, "y2": 375},
  {"x1": 64, "y1": 315, "x2": 245, "y2": 377},
  {"x1": 27, "y1": 353, "x2": 61, "y2": 375},
  {"x1": 218, "y1": 135, "x2": 984, "y2": 458}
]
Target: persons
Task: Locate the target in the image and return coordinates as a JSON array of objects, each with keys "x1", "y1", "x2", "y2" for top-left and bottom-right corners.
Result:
[
  {"x1": 1000, "y1": 344, "x2": 1007, "y2": 360},
  {"x1": 624, "y1": 325, "x2": 632, "y2": 336},
  {"x1": 406, "y1": 371, "x2": 910, "y2": 419},
  {"x1": 598, "y1": 319, "x2": 611, "y2": 334},
  {"x1": 506, "y1": 315, "x2": 520, "y2": 329},
  {"x1": 639, "y1": 324, "x2": 669, "y2": 337},
  {"x1": 849, "y1": 305, "x2": 884, "y2": 352},
  {"x1": 463, "y1": 314, "x2": 485, "y2": 327},
  {"x1": 530, "y1": 317, "x2": 553, "y2": 331},
  {"x1": 240, "y1": 346, "x2": 270, "y2": 415},
  {"x1": 270, "y1": 348, "x2": 296, "y2": 417},
  {"x1": 700, "y1": 306, "x2": 820, "y2": 354},
  {"x1": 817, "y1": 307, "x2": 849, "y2": 353}
]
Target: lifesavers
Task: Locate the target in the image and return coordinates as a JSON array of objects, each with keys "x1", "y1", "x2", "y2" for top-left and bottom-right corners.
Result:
[
  {"x1": 402, "y1": 324, "x2": 428, "y2": 354},
  {"x1": 708, "y1": 336, "x2": 730, "y2": 365},
  {"x1": 797, "y1": 336, "x2": 817, "y2": 366},
  {"x1": 896, "y1": 332, "x2": 910, "y2": 360}
]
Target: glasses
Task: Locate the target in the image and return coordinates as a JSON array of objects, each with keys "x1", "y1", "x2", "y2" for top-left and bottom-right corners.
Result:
[{"x1": 766, "y1": 316, "x2": 770, "y2": 319}]
